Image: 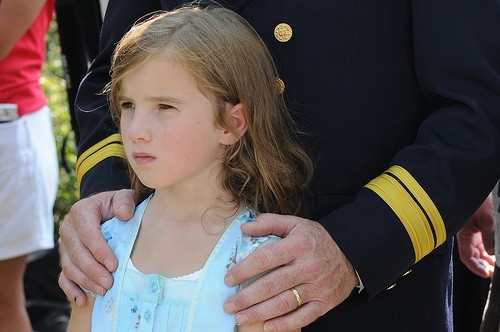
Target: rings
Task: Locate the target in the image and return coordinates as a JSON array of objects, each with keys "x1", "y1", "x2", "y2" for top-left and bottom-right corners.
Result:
[{"x1": 289, "y1": 287, "x2": 303, "y2": 307}]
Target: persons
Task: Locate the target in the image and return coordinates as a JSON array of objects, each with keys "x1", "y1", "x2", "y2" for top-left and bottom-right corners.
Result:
[
  {"x1": 65, "y1": 4, "x2": 315, "y2": 332},
  {"x1": 0, "y1": 0, "x2": 61, "y2": 332},
  {"x1": 456, "y1": 178, "x2": 500, "y2": 332},
  {"x1": 59, "y1": 0, "x2": 500, "y2": 332},
  {"x1": 57, "y1": 0, "x2": 110, "y2": 143}
]
